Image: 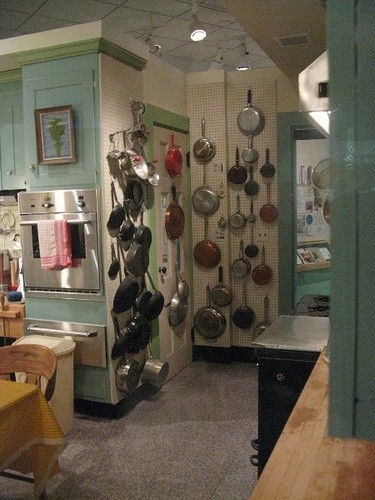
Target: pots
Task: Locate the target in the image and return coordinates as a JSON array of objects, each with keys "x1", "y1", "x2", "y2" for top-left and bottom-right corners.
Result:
[
  {"x1": 259, "y1": 183, "x2": 278, "y2": 223},
  {"x1": 252, "y1": 246, "x2": 272, "y2": 285},
  {"x1": 260, "y1": 149, "x2": 276, "y2": 179},
  {"x1": 194, "y1": 286, "x2": 226, "y2": 343},
  {"x1": 244, "y1": 167, "x2": 259, "y2": 196},
  {"x1": 237, "y1": 89, "x2": 264, "y2": 136},
  {"x1": 233, "y1": 281, "x2": 255, "y2": 329},
  {"x1": 231, "y1": 239, "x2": 251, "y2": 277},
  {"x1": 252, "y1": 296, "x2": 271, "y2": 338},
  {"x1": 242, "y1": 134, "x2": 258, "y2": 164},
  {"x1": 227, "y1": 148, "x2": 247, "y2": 185},
  {"x1": 229, "y1": 195, "x2": 247, "y2": 229},
  {"x1": 108, "y1": 105, "x2": 220, "y2": 394},
  {"x1": 247, "y1": 200, "x2": 255, "y2": 223},
  {"x1": 245, "y1": 230, "x2": 259, "y2": 258},
  {"x1": 211, "y1": 266, "x2": 233, "y2": 307}
]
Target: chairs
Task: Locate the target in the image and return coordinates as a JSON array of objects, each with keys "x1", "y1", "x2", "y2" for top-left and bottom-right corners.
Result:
[{"x1": 0, "y1": 344, "x2": 57, "y2": 403}]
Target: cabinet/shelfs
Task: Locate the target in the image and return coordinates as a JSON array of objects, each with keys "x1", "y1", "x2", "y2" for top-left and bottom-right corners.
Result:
[{"x1": 0, "y1": 69, "x2": 97, "y2": 191}]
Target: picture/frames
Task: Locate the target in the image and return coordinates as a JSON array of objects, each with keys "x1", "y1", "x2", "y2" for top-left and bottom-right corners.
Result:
[{"x1": 34, "y1": 104, "x2": 76, "y2": 164}]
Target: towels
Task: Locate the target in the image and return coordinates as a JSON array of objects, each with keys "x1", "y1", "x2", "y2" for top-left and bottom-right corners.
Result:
[
  {"x1": 37, "y1": 218, "x2": 73, "y2": 271},
  {"x1": 72, "y1": 259, "x2": 81, "y2": 268}
]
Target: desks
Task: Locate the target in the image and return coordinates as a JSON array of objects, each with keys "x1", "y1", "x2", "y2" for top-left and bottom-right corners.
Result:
[{"x1": 0, "y1": 380, "x2": 69, "y2": 500}]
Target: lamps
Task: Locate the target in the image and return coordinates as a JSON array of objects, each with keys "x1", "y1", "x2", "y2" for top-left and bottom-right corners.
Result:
[
  {"x1": 144, "y1": 37, "x2": 161, "y2": 55},
  {"x1": 236, "y1": 43, "x2": 251, "y2": 71},
  {"x1": 207, "y1": 48, "x2": 224, "y2": 70},
  {"x1": 189, "y1": 15, "x2": 206, "y2": 42}
]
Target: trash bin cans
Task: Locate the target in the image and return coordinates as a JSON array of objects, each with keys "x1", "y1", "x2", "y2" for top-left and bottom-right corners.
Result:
[{"x1": 11, "y1": 333, "x2": 77, "y2": 437}]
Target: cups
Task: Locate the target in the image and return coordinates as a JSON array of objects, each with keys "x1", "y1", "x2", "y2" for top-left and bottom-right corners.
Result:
[{"x1": 0, "y1": 284, "x2": 8, "y2": 311}]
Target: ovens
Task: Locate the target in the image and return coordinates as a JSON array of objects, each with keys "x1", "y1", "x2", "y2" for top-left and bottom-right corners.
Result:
[{"x1": 17, "y1": 189, "x2": 108, "y2": 302}]
